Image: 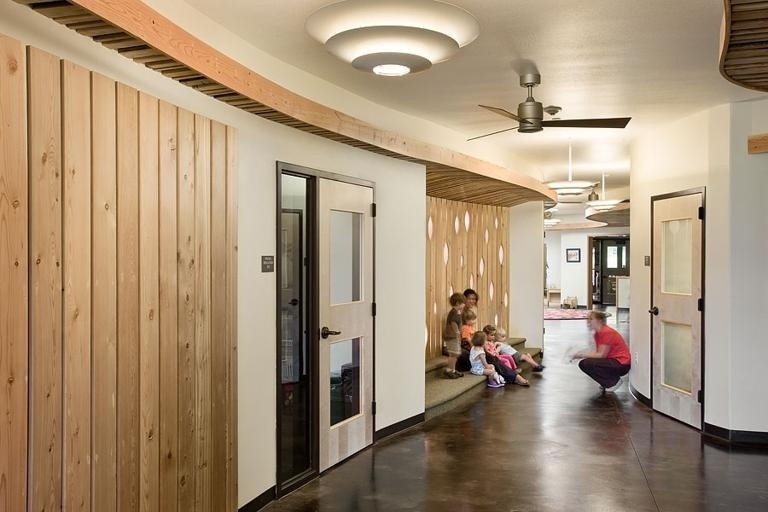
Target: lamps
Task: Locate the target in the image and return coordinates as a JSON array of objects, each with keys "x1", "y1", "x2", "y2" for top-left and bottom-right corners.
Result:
[
  {"x1": 542, "y1": 142, "x2": 593, "y2": 196},
  {"x1": 302, "y1": 0, "x2": 481, "y2": 76},
  {"x1": 586, "y1": 173, "x2": 620, "y2": 211}
]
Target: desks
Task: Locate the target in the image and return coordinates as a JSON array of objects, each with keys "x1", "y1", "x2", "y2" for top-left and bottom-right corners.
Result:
[{"x1": 547, "y1": 288, "x2": 561, "y2": 307}]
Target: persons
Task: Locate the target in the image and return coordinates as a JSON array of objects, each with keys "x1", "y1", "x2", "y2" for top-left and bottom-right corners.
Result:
[
  {"x1": 573, "y1": 311, "x2": 631, "y2": 392},
  {"x1": 443, "y1": 289, "x2": 545, "y2": 387}
]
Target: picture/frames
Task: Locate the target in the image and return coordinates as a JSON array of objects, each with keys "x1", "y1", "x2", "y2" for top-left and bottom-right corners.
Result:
[{"x1": 565, "y1": 248, "x2": 581, "y2": 263}]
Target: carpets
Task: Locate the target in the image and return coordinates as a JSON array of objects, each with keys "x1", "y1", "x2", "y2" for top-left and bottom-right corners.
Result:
[{"x1": 544, "y1": 308, "x2": 611, "y2": 320}]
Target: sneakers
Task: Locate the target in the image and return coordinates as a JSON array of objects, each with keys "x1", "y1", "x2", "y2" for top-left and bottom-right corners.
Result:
[
  {"x1": 531, "y1": 363, "x2": 546, "y2": 373},
  {"x1": 514, "y1": 373, "x2": 531, "y2": 387},
  {"x1": 599, "y1": 376, "x2": 624, "y2": 392},
  {"x1": 487, "y1": 377, "x2": 506, "y2": 388},
  {"x1": 442, "y1": 367, "x2": 465, "y2": 379},
  {"x1": 513, "y1": 366, "x2": 523, "y2": 373}
]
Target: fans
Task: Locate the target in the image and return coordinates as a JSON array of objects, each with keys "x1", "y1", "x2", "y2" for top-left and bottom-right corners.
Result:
[{"x1": 466, "y1": 72, "x2": 632, "y2": 142}]
